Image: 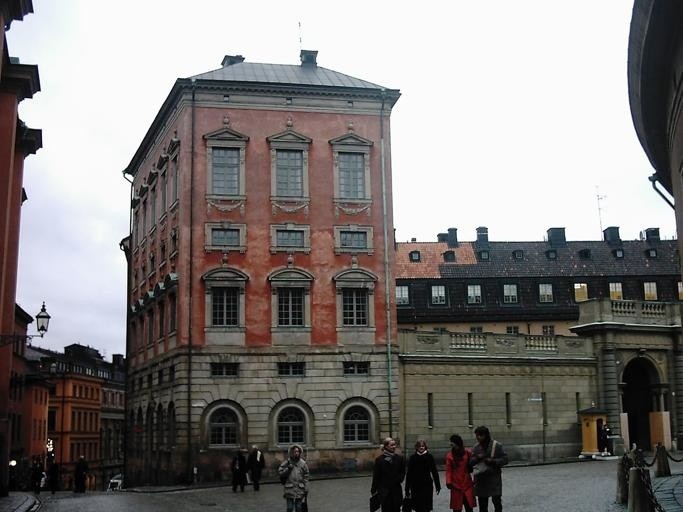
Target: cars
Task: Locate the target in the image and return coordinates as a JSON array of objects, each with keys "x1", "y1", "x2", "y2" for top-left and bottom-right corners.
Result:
[{"x1": 106, "y1": 478, "x2": 121, "y2": 492}]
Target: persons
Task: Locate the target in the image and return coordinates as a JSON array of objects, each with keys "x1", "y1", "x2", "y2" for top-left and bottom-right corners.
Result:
[
  {"x1": 467, "y1": 425, "x2": 509, "y2": 512},
  {"x1": 598, "y1": 423, "x2": 611, "y2": 457},
  {"x1": 276, "y1": 443, "x2": 311, "y2": 511},
  {"x1": 369, "y1": 436, "x2": 405, "y2": 511},
  {"x1": 404, "y1": 437, "x2": 441, "y2": 512},
  {"x1": 230, "y1": 448, "x2": 245, "y2": 493},
  {"x1": 443, "y1": 433, "x2": 479, "y2": 511},
  {"x1": 243, "y1": 444, "x2": 267, "y2": 492},
  {"x1": 70, "y1": 454, "x2": 88, "y2": 494}
]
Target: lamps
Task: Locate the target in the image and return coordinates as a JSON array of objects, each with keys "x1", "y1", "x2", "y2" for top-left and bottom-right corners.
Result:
[{"x1": 0, "y1": 301, "x2": 51, "y2": 348}]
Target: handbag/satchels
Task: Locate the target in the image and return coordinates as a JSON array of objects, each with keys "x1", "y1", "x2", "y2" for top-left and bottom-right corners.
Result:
[
  {"x1": 301, "y1": 502, "x2": 308, "y2": 512},
  {"x1": 370, "y1": 494, "x2": 380, "y2": 512},
  {"x1": 402, "y1": 496, "x2": 412, "y2": 512}
]
